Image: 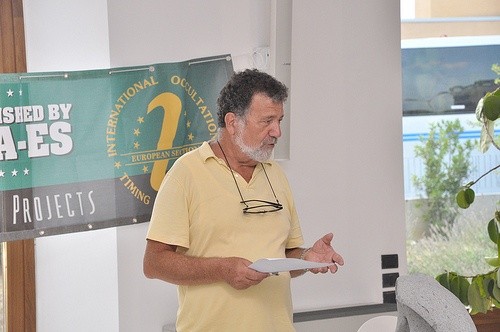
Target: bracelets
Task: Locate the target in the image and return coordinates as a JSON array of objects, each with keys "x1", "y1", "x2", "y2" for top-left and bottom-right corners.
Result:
[{"x1": 299, "y1": 247, "x2": 311, "y2": 273}]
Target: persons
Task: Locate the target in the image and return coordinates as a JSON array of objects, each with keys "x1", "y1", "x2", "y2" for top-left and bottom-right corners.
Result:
[{"x1": 143, "y1": 69, "x2": 344, "y2": 332}]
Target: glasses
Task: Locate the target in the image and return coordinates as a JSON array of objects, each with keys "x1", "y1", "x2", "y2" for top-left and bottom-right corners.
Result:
[{"x1": 240, "y1": 200, "x2": 283, "y2": 213}]
